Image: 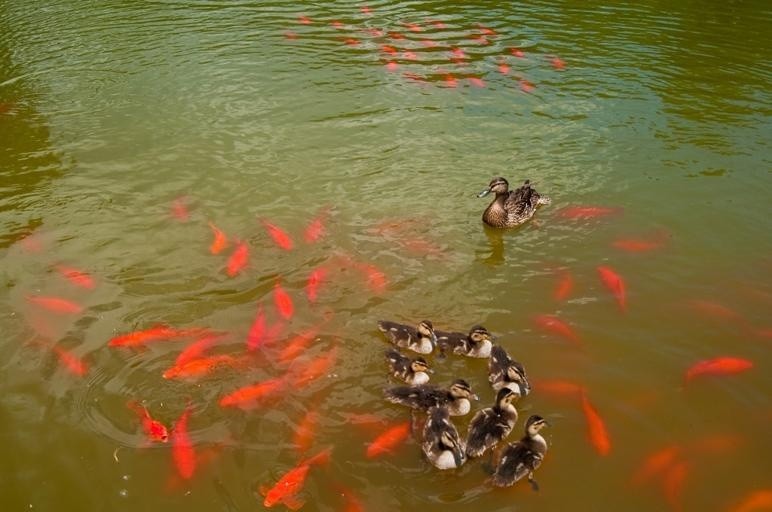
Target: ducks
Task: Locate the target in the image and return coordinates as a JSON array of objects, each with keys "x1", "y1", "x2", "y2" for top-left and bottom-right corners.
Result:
[
  {"x1": 378, "y1": 321, "x2": 552, "y2": 492},
  {"x1": 476, "y1": 176, "x2": 552, "y2": 229}
]
